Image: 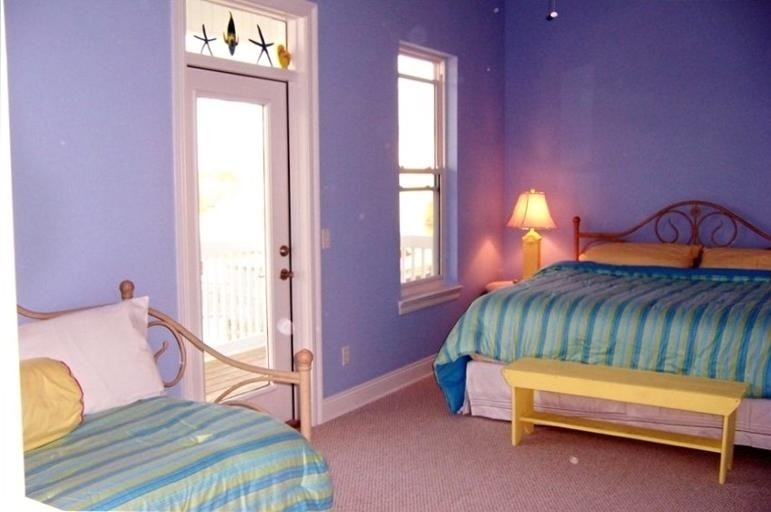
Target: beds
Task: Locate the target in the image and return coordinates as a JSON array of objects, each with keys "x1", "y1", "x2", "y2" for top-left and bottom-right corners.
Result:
[{"x1": 434, "y1": 201, "x2": 771, "y2": 450}]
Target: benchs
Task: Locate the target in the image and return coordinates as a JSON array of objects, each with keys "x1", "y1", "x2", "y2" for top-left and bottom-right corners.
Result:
[{"x1": 501, "y1": 357, "x2": 750, "y2": 485}]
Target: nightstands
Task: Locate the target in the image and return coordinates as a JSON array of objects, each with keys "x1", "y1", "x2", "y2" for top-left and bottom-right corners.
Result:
[{"x1": 485, "y1": 280, "x2": 521, "y2": 292}]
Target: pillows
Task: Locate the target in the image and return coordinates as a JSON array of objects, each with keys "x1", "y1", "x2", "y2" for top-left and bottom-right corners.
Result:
[
  {"x1": 19, "y1": 357, "x2": 84, "y2": 453},
  {"x1": 698, "y1": 246, "x2": 771, "y2": 270},
  {"x1": 578, "y1": 240, "x2": 703, "y2": 269},
  {"x1": 18, "y1": 296, "x2": 168, "y2": 416}
]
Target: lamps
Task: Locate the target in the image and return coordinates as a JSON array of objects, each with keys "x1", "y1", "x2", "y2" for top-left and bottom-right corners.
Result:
[{"x1": 503, "y1": 188, "x2": 557, "y2": 280}]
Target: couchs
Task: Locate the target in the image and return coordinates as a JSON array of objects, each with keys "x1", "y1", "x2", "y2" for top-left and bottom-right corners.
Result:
[{"x1": 17, "y1": 281, "x2": 334, "y2": 512}]
